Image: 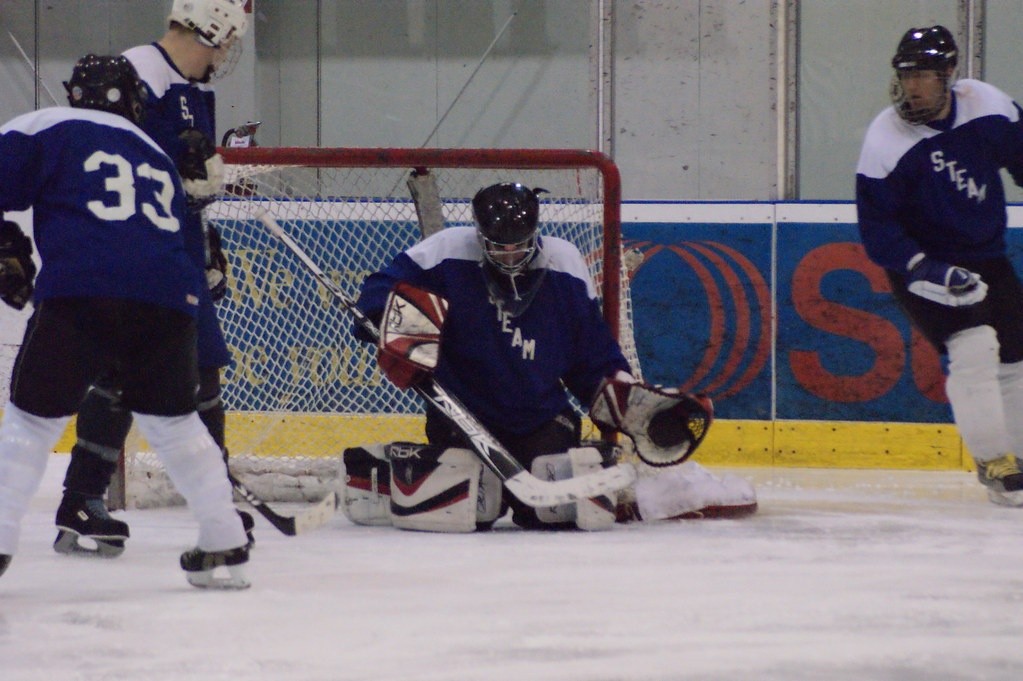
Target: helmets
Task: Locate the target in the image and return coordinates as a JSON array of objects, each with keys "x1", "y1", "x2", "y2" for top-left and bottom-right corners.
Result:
[
  {"x1": 166, "y1": 0, "x2": 249, "y2": 80},
  {"x1": 892, "y1": 25, "x2": 959, "y2": 126},
  {"x1": 472, "y1": 183, "x2": 542, "y2": 275},
  {"x1": 62, "y1": 55, "x2": 144, "y2": 128}
]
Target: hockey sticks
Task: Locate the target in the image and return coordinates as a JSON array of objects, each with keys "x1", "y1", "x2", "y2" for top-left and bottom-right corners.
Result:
[
  {"x1": 252, "y1": 204, "x2": 638, "y2": 509},
  {"x1": 29, "y1": 288, "x2": 341, "y2": 538}
]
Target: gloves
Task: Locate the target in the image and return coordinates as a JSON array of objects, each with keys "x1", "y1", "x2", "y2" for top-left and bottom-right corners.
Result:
[
  {"x1": 902, "y1": 254, "x2": 999, "y2": 316},
  {"x1": 0, "y1": 220, "x2": 38, "y2": 311}
]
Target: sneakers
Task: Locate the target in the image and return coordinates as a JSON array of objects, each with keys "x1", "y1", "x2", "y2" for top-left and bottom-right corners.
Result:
[
  {"x1": 179, "y1": 542, "x2": 252, "y2": 588},
  {"x1": 53, "y1": 472, "x2": 130, "y2": 559},
  {"x1": 234, "y1": 506, "x2": 255, "y2": 546},
  {"x1": 975, "y1": 451, "x2": 1023, "y2": 508}
]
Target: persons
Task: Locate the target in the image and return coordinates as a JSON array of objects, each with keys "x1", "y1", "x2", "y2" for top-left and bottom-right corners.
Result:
[
  {"x1": 855, "y1": 26, "x2": 1022, "y2": 508},
  {"x1": 338, "y1": 182, "x2": 758, "y2": 532},
  {"x1": 0, "y1": 52, "x2": 254, "y2": 589},
  {"x1": 50, "y1": 0, "x2": 251, "y2": 558}
]
